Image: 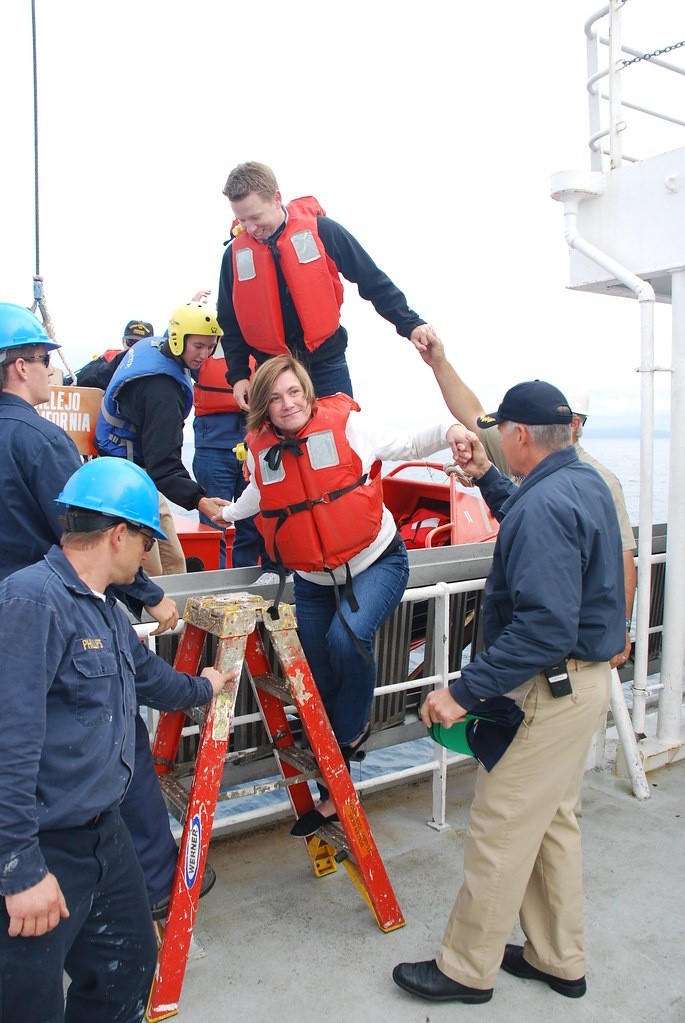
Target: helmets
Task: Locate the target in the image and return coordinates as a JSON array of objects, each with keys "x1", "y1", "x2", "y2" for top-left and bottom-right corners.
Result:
[
  {"x1": 56, "y1": 457, "x2": 169, "y2": 542},
  {"x1": 168, "y1": 301, "x2": 224, "y2": 358},
  {"x1": 557, "y1": 383, "x2": 590, "y2": 416},
  {"x1": 0, "y1": 301, "x2": 61, "y2": 351}
]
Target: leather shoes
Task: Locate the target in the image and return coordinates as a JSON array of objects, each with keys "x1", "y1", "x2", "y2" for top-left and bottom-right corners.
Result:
[
  {"x1": 392, "y1": 959, "x2": 493, "y2": 1005},
  {"x1": 501, "y1": 944, "x2": 586, "y2": 998}
]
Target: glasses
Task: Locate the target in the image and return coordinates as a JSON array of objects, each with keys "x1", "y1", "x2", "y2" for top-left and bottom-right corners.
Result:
[
  {"x1": 2, "y1": 355, "x2": 50, "y2": 368},
  {"x1": 125, "y1": 339, "x2": 136, "y2": 347},
  {"x1": 102, "y1": 522, "x2": 155, "y2": 552}
]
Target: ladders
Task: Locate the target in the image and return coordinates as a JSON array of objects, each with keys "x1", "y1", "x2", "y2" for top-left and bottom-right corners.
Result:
[{"x1": 144, "y1": 591, "x2": 405, "y2": 1023}]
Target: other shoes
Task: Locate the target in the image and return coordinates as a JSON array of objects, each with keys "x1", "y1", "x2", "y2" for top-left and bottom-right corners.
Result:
[
  {"x1": 342, "y1": 726, "x2": 371, "y2": 762},
  {"x1": 148, "y1": 863, "x2": 216, "y2": 920},
  {"x1": 290, "y1": 808, "x2": 341, "y2": 838}
]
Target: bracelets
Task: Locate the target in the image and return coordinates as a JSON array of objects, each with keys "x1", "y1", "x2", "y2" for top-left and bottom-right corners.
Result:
[{"x1": 624, "y1": 618, "x2": 631, "y2": 629}]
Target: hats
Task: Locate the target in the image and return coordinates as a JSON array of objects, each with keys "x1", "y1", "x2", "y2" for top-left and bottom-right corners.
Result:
[
  {"x1": 426, "y1": 695, "x2": 525, "y2": 773},
  {"x1": 122, "y1": 320, "x2": 154, "y2": 341},
  {"x1": 477, "y1": 380, "x2": 572, "y2": 429}
]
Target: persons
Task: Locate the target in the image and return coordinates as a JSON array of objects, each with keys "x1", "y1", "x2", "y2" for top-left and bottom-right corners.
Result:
[
  {"x1": 0, "y1": 301, "x2": 217, "y2": 918},
  {"x1": 217, "y1": 158, "x2": 442, "y2": 405},
  {"x1": 207, "y1": 349, "x2": 472, "y2": 839},
  {"x1": 61, "y1": 320, "x2": 154, "y2": 390},
  {"x1": 164, "y1": 286, "x2": 264, "y2": 571},
  {"x1": 422, "y1": 326, "x2": 638, "y2": 670},
  {"x1": 0, "y1": 448, "x2": 239, "y2": 1023},
  {"x1": 93, "y1": 301, "x2": 236, "y2": 581},
  {"x1": 390, "y1": 377, "x2": 627, "y2": 1007}
]
